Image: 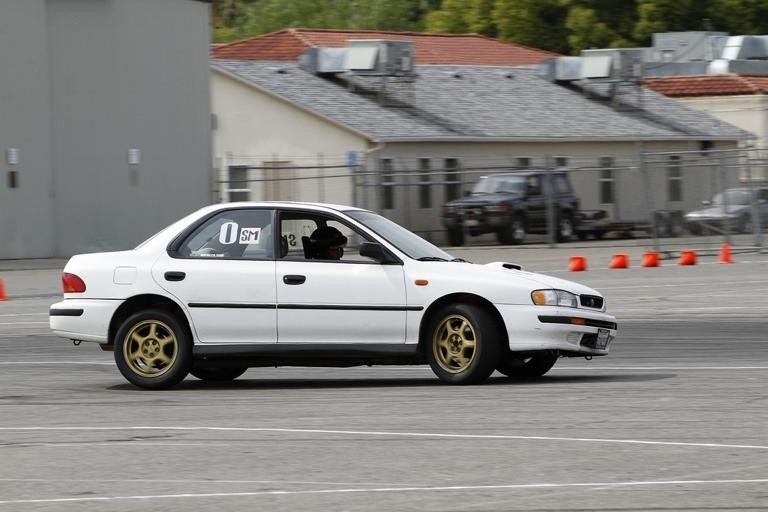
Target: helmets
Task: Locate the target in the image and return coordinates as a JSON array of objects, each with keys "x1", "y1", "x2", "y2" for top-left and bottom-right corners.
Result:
[{"x1": 309, "y1": 226, "x2": 347, "y2": 259}]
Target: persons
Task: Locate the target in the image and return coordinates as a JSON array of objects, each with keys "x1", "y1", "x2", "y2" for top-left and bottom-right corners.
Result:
[{"x1": 308, "y1": 225, "x2": 351, "y2": 261}]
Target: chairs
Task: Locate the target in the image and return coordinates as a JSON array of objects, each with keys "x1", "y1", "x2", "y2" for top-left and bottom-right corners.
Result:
[{"x1": 300, "y1": 236, "x2": 311, "y2": 260}]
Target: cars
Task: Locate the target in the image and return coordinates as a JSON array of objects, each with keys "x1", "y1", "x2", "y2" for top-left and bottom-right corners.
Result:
[
  {"x1": 49, "y1": 201, "x2": 617, "y2": 391},
  {"x1": 684, "y1": 187, "x2": 768, "y2": 235}
]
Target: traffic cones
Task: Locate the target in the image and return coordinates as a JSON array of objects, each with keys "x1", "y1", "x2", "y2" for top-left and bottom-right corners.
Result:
[
  {"x1": 568, "y1": 244, "x2": 734, "y2": 270},
  {"x1": 0, "y1": 279, "x2": 6, "y2": 300}
]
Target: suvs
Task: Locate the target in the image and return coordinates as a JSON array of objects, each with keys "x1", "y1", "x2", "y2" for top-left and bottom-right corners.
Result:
[{"x1": 441, "y1": 169, "x2": 579, "y2": 247}]
